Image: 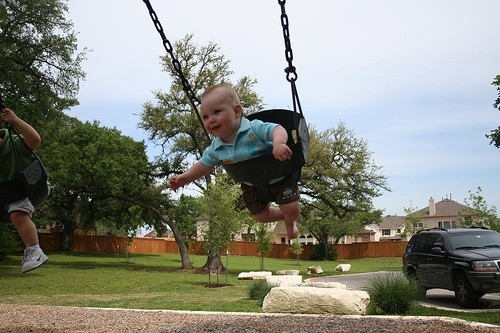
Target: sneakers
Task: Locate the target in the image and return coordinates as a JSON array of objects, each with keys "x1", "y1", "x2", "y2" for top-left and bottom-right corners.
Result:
[{"x1": 21, "y1": 244, "x2": 49, "y2": 273}]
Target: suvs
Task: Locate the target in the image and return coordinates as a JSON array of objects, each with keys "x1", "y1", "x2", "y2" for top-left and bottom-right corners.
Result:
[{"x1": 401, "y1": 225, "x2": 500, "y2": 308}]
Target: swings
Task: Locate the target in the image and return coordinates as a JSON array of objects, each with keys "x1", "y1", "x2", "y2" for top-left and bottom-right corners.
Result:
[
  {"x1": 0, "y1": 119, "x2": 52, "y2": 221},
  {"x1": 142, "y1": 0, "x2": 312, "y2": 185}
]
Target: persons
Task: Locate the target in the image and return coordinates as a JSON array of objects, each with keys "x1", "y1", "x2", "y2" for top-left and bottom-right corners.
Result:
[
  {"x1": 0, "y1": 108, "x2": 50, "y2": 273},
  {"x1": 167, "y1": 81, "x2": 302, "y2": 241}
]
